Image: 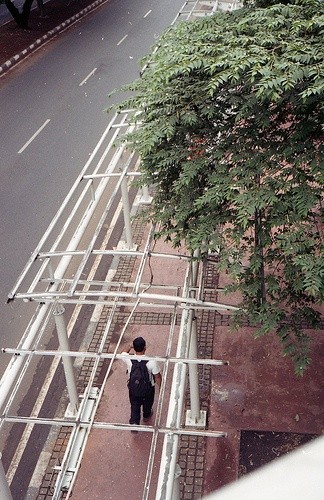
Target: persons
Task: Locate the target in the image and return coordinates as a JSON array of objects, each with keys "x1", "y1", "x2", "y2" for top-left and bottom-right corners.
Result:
[{"x1": 119, "y1": 336, "x2": 161, "y2": 431}]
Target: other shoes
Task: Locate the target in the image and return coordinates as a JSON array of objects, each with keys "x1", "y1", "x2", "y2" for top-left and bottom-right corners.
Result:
[{"x1": 143, "y1": 409, "x2": 154, "y2": 421}]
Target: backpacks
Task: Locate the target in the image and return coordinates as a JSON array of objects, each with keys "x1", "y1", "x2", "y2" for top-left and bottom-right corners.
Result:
[{"x1": 127, "y1": 359, "x2": 152, "y2": 401}]
